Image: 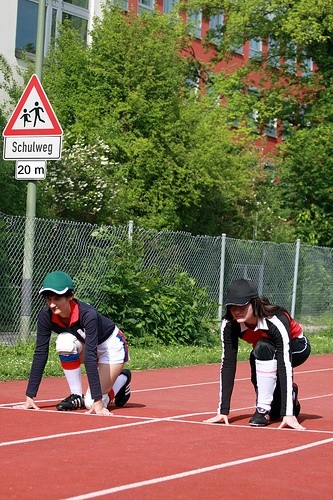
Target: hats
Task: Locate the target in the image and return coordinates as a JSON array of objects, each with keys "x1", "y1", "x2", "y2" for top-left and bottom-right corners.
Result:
[
  {"x1": 223, "y1": 278, "x2": 258, "y2": 306},
  {"x1": 39, "y1": 270, "x2": 74, "y2": 294}
]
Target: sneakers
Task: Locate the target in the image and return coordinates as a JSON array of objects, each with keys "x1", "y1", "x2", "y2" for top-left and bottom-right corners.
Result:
[
  {"x1": 291, "y1": 383, "x2": 300, "y2": 418},
  {"x1": 248, "y1": 409, "x2": 270, "y2": 425},
  {"x1": 115, "y1": 369, "x2": 132, "y2": 408},
  {"x1": 56, "y1": 393, "x2": 82, "y2": 410}
]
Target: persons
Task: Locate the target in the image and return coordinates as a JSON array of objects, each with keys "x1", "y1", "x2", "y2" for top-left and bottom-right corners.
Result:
[
  {"x1": 204, "y1": 277, "x2": 310, "y2": 430},
  {"x1": 13, "y1": 272, "x2": 131, "y2": 416}
]
